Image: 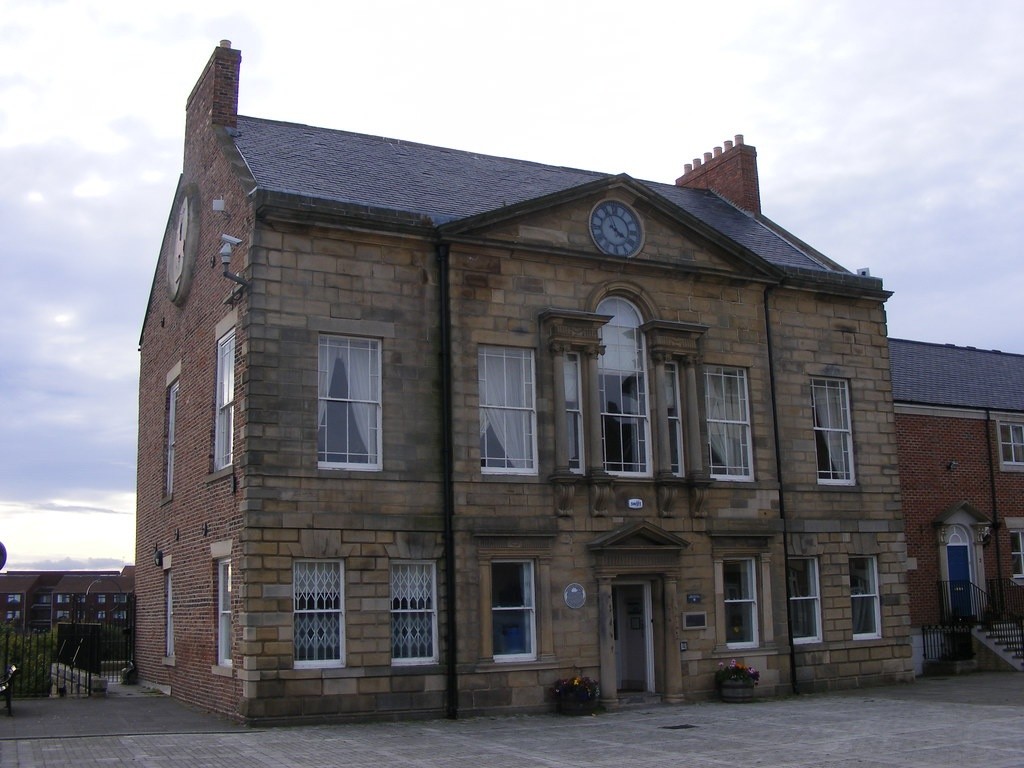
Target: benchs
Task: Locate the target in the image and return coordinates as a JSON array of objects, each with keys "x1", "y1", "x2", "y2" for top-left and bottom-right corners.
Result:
[{"x1": 0, "y1": 664, "x2": 19, "y2": 716}]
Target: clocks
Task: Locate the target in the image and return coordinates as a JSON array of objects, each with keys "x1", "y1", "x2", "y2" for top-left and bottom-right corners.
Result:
[{"x1": 590, "y1": 198, "x2": 646, "y2": 257}]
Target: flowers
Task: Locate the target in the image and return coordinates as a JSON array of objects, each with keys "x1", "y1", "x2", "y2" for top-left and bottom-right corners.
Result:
[
  {"x1": 552, "y1": 676, "x2": 600, "y2": 703},
  {"x1": 716, "y1": 658, "x2": 759, "y2": 687}
]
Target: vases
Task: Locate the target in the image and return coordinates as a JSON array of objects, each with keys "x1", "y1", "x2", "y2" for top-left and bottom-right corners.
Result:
[
  {"x1": 721, "y1": 679, "x2": 755, "y2": 702},
  {"x1": 557, "y1": 704, "x2": 597, "y2": 716}
]
